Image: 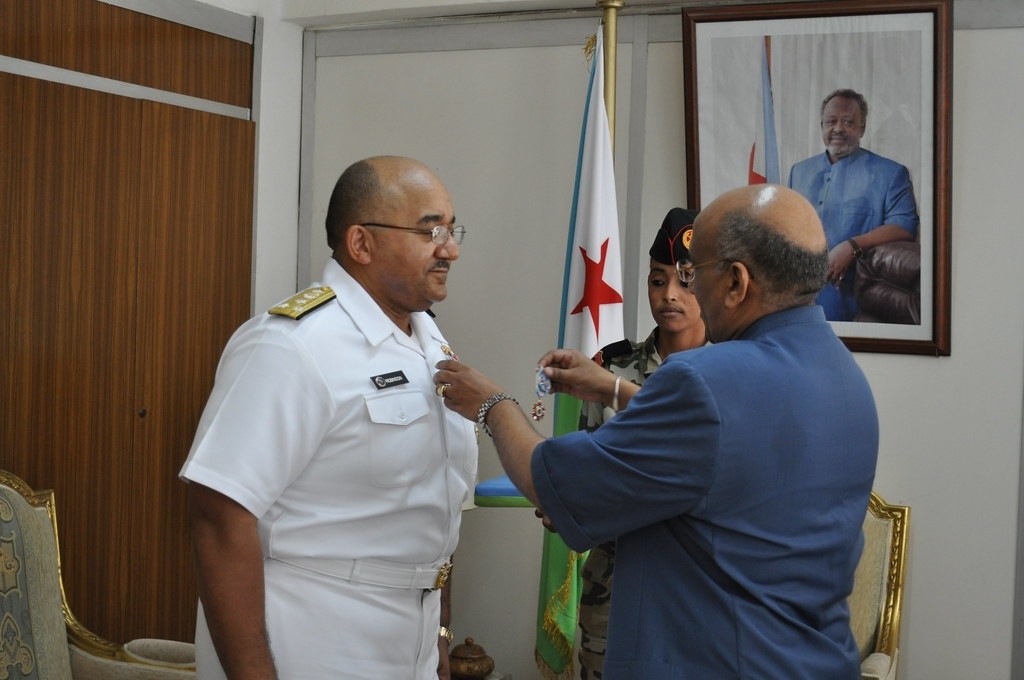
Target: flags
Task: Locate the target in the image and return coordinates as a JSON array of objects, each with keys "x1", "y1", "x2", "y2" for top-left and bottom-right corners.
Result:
[
  {"x1": 534, "y1": 17, "x2": 624, "y2": 678},
  {"x1": 748, "y1": 36, "x2": 780, "y2": 184}
]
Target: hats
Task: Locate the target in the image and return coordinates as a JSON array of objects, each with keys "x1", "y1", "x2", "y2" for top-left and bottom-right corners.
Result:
[{"x1": 649, "y1": 207, "x2": 697, "y2": 264}]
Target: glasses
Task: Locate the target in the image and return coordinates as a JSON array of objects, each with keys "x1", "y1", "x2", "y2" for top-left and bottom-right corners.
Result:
[
  {"x1": 675, "y1": 259, "x2": 753, "y2": 283},
  {"x1": 361, "y1": 222, "x2": 464, "y2": 245}
]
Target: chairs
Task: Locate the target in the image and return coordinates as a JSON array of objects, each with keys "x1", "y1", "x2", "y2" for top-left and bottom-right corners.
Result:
[
  {"x1": 0, "y1": 469, "x2": 197, "y2": 680},
  {"x1": 846, "y1": 490, "x2": 910, "y2": 680}
]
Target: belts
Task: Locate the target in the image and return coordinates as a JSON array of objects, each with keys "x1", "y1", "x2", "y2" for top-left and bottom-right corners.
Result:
[{"x1": 269, "y1": 560, "x2": 452, "y2": 590}]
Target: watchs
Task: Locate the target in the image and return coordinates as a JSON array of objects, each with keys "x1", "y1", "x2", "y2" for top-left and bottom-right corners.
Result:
[{"x1": 848, "y1": 238, "x2": 863, "y2": 258}]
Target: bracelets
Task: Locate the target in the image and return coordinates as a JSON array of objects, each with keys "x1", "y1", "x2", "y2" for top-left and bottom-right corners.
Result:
[
  {"x1": 439, "y1": 626, "x2": 453, "y2": 644},
  {"x1": 613, "y1": 376, "x2": 621, "y2": 411},
  {"x1": 478, "y1": 392, "x2": 520, "y2": 437}
]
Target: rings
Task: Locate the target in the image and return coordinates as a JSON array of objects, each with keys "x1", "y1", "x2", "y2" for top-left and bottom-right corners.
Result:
[
  {"x1": 839, "y1": 277, "x2": 845, "y2": 281},
  {"x1": 437, "y1": 385, "x2": 448, "y2": 397}
]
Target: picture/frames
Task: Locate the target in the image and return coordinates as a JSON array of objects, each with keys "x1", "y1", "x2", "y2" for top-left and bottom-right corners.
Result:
[{"x1": 680, "y1": 0, "x2": 953, "y2": 358}]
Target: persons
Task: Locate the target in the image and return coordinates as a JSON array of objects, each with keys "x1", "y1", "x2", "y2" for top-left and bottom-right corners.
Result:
[
  {"x1": 578, "y1": 208, "x2": 712, "y2": 680},
  {"x1": 178, "y1": 155, "x2": 477, "y2": 680},
  {"x1": 434, "y1": 184, "x2": 881, "y2": 680},
  {"x1": 786, "y1": 89, "x2": 921, "y2": 322}
]
